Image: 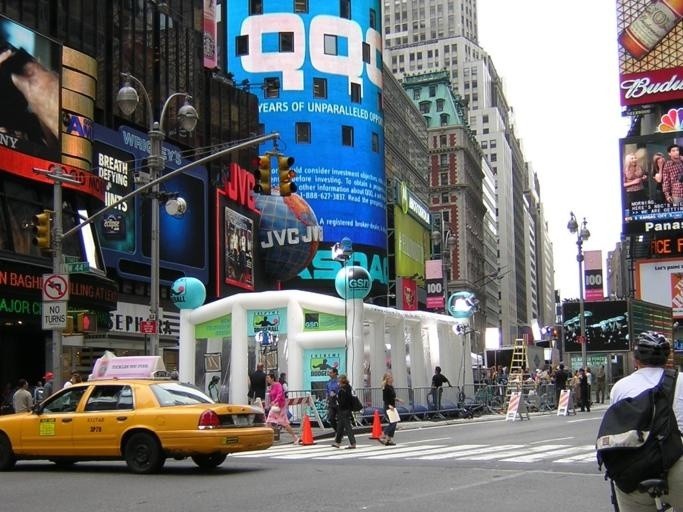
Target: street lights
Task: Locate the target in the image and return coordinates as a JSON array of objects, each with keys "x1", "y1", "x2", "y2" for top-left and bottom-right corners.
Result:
[
  {"x1": 567, "y1": 211, "x2": 590, "y2": 366},
  {"x1": 114, "y1": 67, "x2": 200, "y2": 352}
]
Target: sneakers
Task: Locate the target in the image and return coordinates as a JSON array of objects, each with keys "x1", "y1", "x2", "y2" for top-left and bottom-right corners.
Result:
[
  {"x1": 378, "y1": 435, "x2": 396, "y2": 445},
  {"x1": 345, "y1": 447, "x2": 355, "y2": 449},
  {"x1": 332, "y1": 444, "x2": 339, "y2": 448}
]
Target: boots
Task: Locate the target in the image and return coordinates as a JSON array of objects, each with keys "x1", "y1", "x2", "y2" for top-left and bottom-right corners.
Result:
[{"x1": 292, "y1": 434, "x2": 299, "y2": 445}]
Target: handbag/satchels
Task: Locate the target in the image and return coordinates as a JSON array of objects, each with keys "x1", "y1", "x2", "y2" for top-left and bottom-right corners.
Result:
[
  {"x1": 352, "y1": 394, "x2": 363, "y2": 412},
  {"x1": 271, "y1": 406, "x2": 281, "y2": 413},
  {"x1": 596, "y1": 367, "x2": 683, "y2": 494}
]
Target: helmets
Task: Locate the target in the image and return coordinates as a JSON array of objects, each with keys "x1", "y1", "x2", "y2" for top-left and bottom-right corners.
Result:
[{"x1": 633, "y1": 330, "x2": 670, "y2": 366}]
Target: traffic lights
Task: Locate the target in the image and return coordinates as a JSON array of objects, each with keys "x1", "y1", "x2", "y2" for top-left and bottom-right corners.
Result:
[
  {"x1": 253, "y1": 155, "x2": 269, "y2": 194},
  {"x1": 279, "y1": 156, "x2": 297, "y2": 194}
]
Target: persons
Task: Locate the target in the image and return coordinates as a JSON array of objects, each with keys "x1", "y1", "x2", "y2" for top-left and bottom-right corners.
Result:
[
  {"x1": 2, "y1": 371, "x2": 82, "y2": 414},
  {"x1": 431, "y1": 366, "x2": 451, "y2": 411},
  {"x1": 623, "y1": 144, "x2": 683, "y2": 207},
  {"x1": 378, "y1": 372, "x2": 404, "y2": 446},
  {"x1": 554, "y1": 364, "x2": 605, "y2": 412},
  {"x1": 248, "y1": 363, "x2": 356, "y2": 450},
  {"x1": 610, "y1": 330, "x2": 683, "y2": 512},
  {"x1": 208, "y1": 376, "x2": 221, "y2": 403}
]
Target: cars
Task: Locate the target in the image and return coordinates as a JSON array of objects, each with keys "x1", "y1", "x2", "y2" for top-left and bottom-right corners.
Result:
[{"x1": 0, "y1": 352, "x2": 273, "y2": 472}]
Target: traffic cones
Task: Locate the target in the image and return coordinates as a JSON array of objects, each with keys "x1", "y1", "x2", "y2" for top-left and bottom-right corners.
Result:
[
  {"x1": 369, "y1": 409, "x2": 383, "y2": 439},
  {"x1": 299, "y1": 413, "x2": 317, "y2": 445}
]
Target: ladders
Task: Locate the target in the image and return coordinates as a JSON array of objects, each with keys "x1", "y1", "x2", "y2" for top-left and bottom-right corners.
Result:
[{"x1": 501, "y1": 339, "x2": 527, "y2": 413}]
